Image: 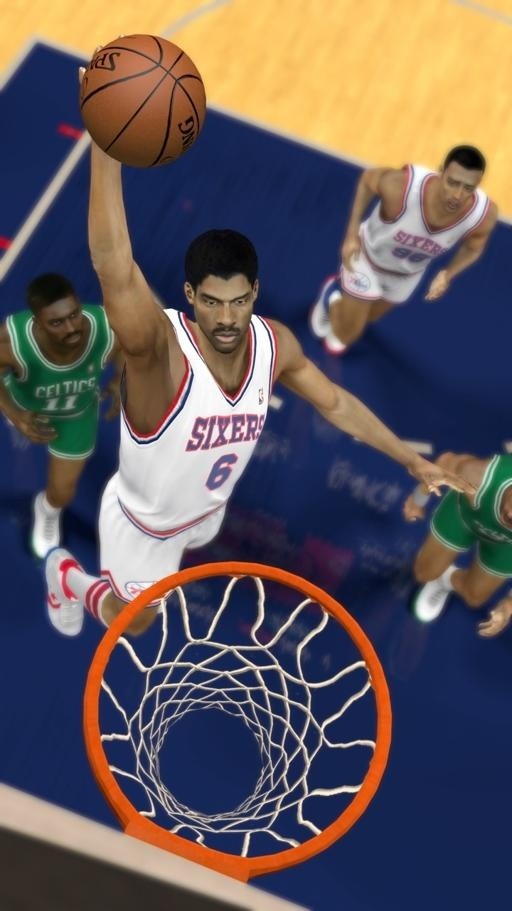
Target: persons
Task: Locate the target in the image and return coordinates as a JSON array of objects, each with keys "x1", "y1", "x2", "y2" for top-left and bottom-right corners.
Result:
[
  {"x1": 305, "y1": 142, "x2": 504, "y2": 360},
  {"x1": 398, "y1": 449, "x2": 511, "y2": 641},
  {"x1": 0, "y1": 270, "x2": 130, "y2": 563},
  {"x1": 40, "y1": 46, "x2": 484, "y2": 642}
]
